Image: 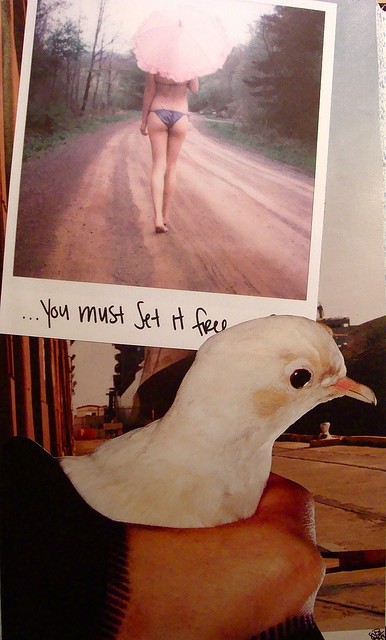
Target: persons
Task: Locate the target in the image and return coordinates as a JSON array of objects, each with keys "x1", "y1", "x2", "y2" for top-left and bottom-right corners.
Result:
[
  {"x1": 1, "y1": 436, "x2": 327, "y2": 639},
  {"x1": 140, "y1": 73, "x2": 200, "y2": 234}
]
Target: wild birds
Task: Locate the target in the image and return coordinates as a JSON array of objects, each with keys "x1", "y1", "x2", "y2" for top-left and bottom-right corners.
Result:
[{"x1": 59, "y1": 316, "x2": 376, "y2": 529}]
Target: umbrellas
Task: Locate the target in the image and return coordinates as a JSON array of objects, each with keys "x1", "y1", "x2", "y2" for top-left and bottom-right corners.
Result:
[{"x1": 130, "y1": 5, "x2": 233, "y2": 83}]
